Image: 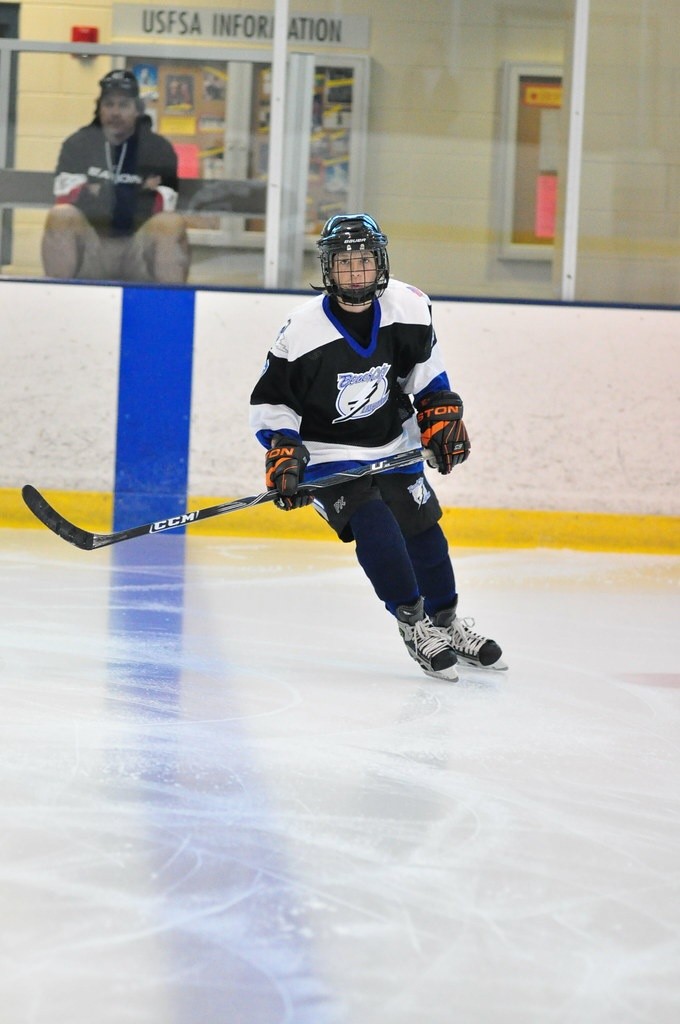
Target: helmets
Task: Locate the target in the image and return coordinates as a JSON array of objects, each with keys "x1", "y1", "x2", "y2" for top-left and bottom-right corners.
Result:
[{"x1": 308, "y1": 213, "x2": 390, "y2": 306}]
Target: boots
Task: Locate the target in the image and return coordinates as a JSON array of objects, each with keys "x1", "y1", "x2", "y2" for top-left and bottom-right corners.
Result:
[
  {"x1": 395, "y1": 596, "x2": 460, "y2": 682},
  {"x1": 429, "y1": 593, "x2": 509, "y2": 672}
]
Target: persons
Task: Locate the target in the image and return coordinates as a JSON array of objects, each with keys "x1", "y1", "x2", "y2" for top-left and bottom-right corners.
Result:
[
  {"x1": 39, "y1": 70, "x2": 192, "y2": 288},
  {"x1": 246, "y1": 211, "x2": 503, "y2": 671}
]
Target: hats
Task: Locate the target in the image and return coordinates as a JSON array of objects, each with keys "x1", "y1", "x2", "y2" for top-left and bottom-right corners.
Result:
[{"x1": 99, "y1": 70, "x2": 138, "y2": 96}]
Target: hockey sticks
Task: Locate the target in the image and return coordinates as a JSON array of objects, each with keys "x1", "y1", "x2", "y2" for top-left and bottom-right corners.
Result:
[{"x1": 22, "y1": 447, "x2": 435, "y2": 550}]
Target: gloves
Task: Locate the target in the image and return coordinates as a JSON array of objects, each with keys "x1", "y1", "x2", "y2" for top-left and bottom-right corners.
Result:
[
  {"x1": 414, "y1": 389, "x2": 471, "y2": 475},
  {"x1": 265, "y1": 434, "x2": 314, "y2": 512}
]
